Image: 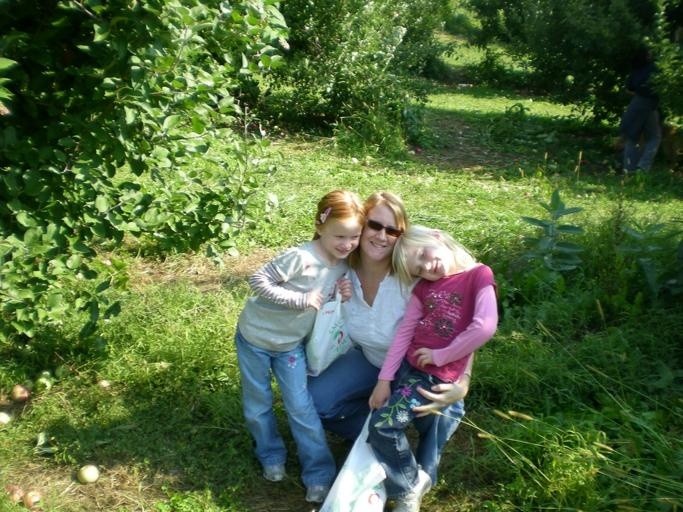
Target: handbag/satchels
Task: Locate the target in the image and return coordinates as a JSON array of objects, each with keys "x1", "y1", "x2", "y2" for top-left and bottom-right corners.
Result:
[{"x1": 303, "y1": 268, "x2": 354, "y2": 377}]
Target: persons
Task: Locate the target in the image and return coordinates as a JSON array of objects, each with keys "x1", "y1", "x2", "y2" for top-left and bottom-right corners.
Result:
[
  {"x1": 363, "y1": 222, "x2": 498, "y2": 512},
  {"x1": 235, "y1": 188, "x2": 367, "y2": 503},
  {"x1": 616, "y1": 39, "x2": 663, "y2": 175},
  {"x1": 303, "y1": 188, "x2": 465, "y2": 490}
]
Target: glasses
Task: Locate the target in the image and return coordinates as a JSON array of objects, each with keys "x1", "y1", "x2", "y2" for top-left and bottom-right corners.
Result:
[{"x1": 365, "y1": 218, "x2": 402, "y2": 238}]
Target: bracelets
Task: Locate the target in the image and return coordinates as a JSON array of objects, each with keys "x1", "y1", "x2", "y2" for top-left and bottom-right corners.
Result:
[{"x1": 464, "y1": 372, "x2": 471, "y2": 380}]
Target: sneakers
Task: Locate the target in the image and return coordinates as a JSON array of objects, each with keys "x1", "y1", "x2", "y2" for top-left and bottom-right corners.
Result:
[
  {"x1": 263, "y1": 465, "x2": 287, "y2": 483},
  {"x1": 392, "y1": 464, "x2": 432, "y2": 512},
  {"x1": 305, "y1": 483, "x2": 329, "y2": 505}
]
